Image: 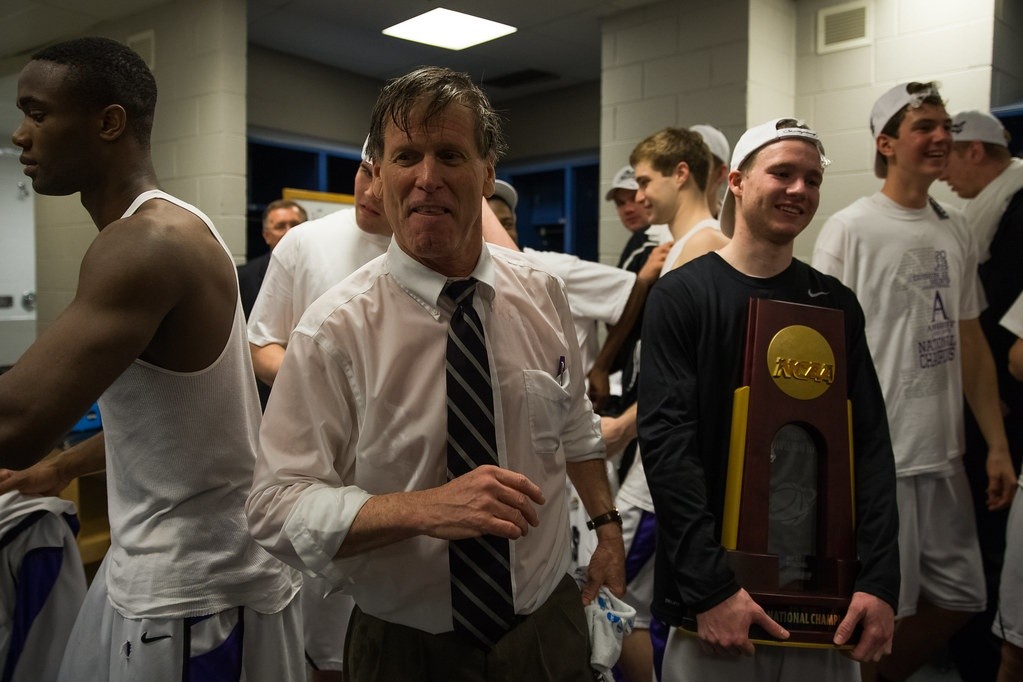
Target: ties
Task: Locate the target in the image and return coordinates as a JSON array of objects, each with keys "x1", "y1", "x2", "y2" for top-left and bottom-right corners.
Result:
[{"x1": 440, "y1": 278, "x2": 517, "y2": 657}]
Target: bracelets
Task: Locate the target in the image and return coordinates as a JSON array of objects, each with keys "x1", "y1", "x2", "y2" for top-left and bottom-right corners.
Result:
[{"x1": 586, "y1": 508, "x2": 624, "y2": 532}]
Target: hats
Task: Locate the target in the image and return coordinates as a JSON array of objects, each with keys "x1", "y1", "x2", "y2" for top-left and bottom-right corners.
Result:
[
  {"x1": 718, "y1": 118, "x2": 825, "y2": 238},
  {"x1": 603, "y1": 166, "x2": 638, "y2": 202},
  {"x1": 690, "y1": 124, "x2": 734, "y2": 172},
  {"x1": 485, "y1": 178, "x2": 517, "y2": 214},
  {"x1": 870, "y1": 82, "x2": 942, "y2": 178},
  {"x1": 949, "y1": 110, "x2": 1006, "y2": 146}
]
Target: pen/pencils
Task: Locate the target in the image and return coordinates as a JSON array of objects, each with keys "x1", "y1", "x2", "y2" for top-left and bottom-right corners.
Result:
[{"x1": 559, "y1": 356, "x2": 565, "y2": 386}]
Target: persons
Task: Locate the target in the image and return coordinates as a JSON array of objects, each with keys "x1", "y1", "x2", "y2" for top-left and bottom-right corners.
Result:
[
  {"x1": 937, "y1": 110, "x2": 1023, "y2": 682},
  {"x1": 230, "y1": 200, "x2": 308, "y2": 418},
  {"x1": 0, "y1": 363, "x2": 88, "y2": 682},
  {"x1": 811, "y1": 83, "x2": 1016, "y2": 682},
  {"x1": 991, "y1": 289, "x2": 1023, "y2": 682},
  {"x1": 592, "y1": 129, "x2": 741, "y2": 682},
  {"x1": 635, "y1": 118, "x2": 898, "y2": 682},
  {"x1": 606, "y1": 165, "x2": 671, "y2": 418},
  {"x1": 0, "y1": 37, "x2": 305, "y2": 682},
  {"x1": 249, "y1": 130, "x2": 522, "y2": 386},
  {"x1": 472, "y1": 175, "x2": 647, "y2": 570},
  {"x1": 684, "y1": 123, "x2": 730, "y2": 225},
  {"x1": 243, "y1": 68, "x2": 627, "y2": 682}
]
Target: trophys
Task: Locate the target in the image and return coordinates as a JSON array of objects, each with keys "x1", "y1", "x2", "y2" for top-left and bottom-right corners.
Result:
[{"x1": 724, "y1": 296, "x2": 861, "y2": 648}]
